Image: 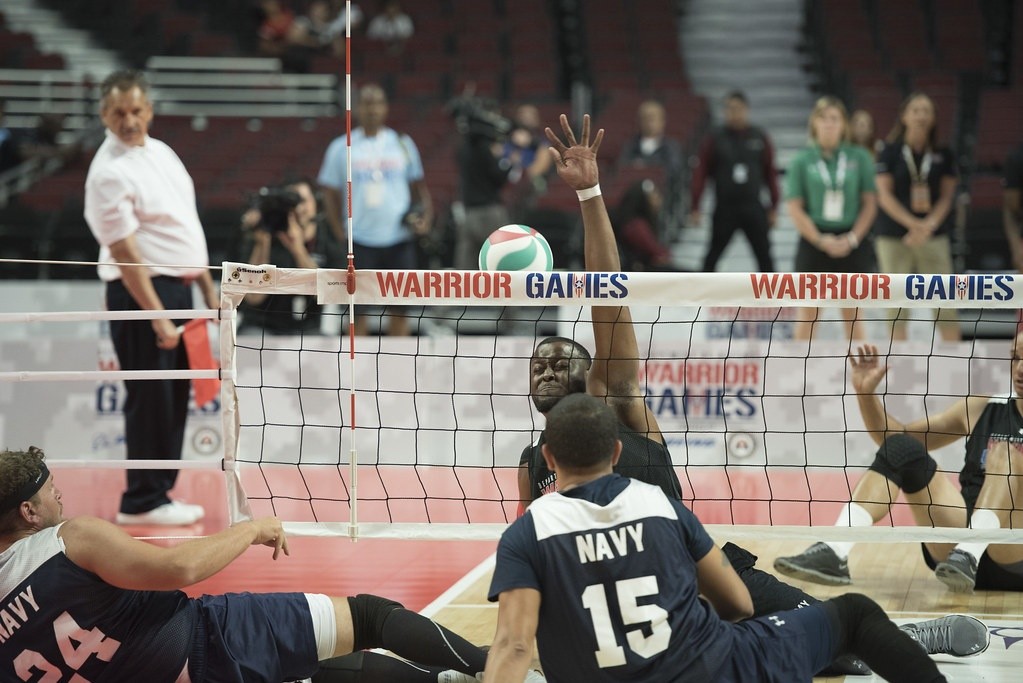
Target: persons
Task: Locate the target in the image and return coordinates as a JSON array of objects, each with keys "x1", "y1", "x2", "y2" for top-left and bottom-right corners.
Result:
[
  {"x1": 0, "y1": 445, "x2": 548, "y2": 683},
  {"x1": 83, "y1": 70, "x2": 223, "y2": 526},
  {"x1": 774, "y1": 313, "x2": 1023, "y2": 592},
  {"x1": 481, "y1": 394, "x2": 950, "y2": 683},
  {"x1": 518, "y1": 113, "x2": 990, "y2": 679},
  {"x1": 231, "y1": 0, "x2": 1023, "y2": 340}
]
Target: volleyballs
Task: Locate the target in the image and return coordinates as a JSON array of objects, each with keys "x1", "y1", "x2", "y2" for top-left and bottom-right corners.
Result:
[{"x1": 478, "y1": 222, "x2": 555, "y2": 273}]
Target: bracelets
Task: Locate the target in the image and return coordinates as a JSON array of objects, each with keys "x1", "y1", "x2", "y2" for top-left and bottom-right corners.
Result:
[{"x1": 576, "y1": 183, "x2": 602, "y2": 201}]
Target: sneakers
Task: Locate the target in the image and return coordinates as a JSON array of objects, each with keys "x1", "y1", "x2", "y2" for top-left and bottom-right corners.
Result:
[
  {"x1": 117, "y1": 500, "x2": 204, "y2": 525},
  {"x1": 898, "y1": 615, "x2": 989, "y2": 658},
  {"x1": 774, "y1": 542, "x2": 850, "y2": 585},
  {"x1": 935, "y1": 549, "x2": 977, "y2": 595}
]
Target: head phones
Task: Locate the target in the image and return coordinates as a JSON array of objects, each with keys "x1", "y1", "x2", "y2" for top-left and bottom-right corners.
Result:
[{"x1": 278, "y1": 175, "x2": 327, "y2": 222}]
